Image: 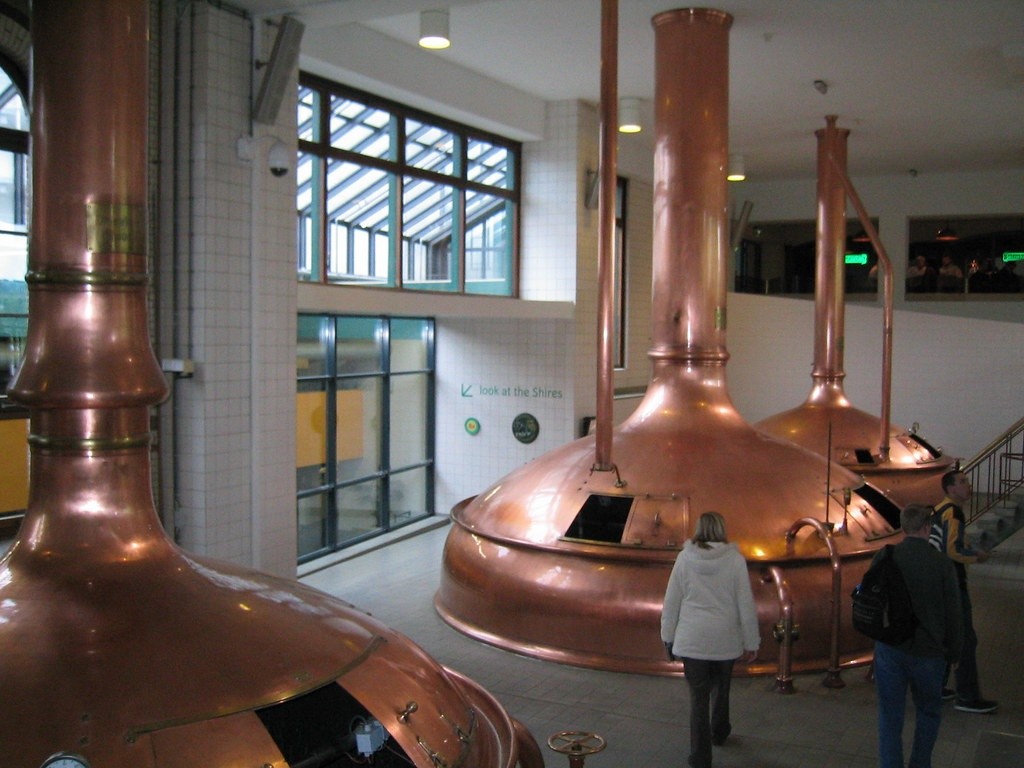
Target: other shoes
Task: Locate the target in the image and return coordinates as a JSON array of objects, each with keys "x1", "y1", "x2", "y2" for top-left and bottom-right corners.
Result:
[{"x1": 712, "y1": 724, "x2": 731, "y2": 745}]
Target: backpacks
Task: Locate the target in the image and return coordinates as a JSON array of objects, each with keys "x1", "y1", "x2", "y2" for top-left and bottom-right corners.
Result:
[{"x1": 851, "y1": 544, "x2": 918, "y2": 648}]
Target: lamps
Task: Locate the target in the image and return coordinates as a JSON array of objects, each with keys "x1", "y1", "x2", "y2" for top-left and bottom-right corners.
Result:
[
  {"x1": 418, "y1": 9, "x2": 450, "y2": 49},
  {"x1": 935, "y1": 222, "x2": 960, "y2": 241},
  {"x1": 237, "y1": 134, "x2": 291, "y2": 176},
  {"x1": 618, "y1": 97, "x2": 646, "y2": 133},
  {"x1": 851, "y1": 230, "x2": 873, "y2": 242},
  {"x1": 727, "y1": 154, "x2": 746, "y2": 180}
]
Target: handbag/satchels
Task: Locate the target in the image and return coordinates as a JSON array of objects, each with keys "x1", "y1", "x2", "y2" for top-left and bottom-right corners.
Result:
[{"x1": 928, "y1": 503, "x2": 960, "y2": 554}]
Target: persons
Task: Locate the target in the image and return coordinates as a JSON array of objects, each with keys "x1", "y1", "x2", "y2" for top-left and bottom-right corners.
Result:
[
  {"x1": 852, "y1": 503, "x2": 964, "y2": 768},
  {"x1": 929, "y1": 470, "x2": 999, "y2": 712},
  {"x1": 660, "y1": 512, "x2": 761, "y2": 768}
]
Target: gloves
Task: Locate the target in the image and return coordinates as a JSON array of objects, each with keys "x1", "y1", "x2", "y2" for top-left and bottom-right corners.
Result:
[{"x1": 664, "y1": 642, "x2": 675, "y2": 661}]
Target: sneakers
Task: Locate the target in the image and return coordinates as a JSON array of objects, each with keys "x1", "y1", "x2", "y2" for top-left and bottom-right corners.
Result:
[
  {"x1": 941, "y1": 688, "x2": 959, "y2": 699},
  {"x1": 953, "y1": 696, "x2": 999, "y2": 712}
]
